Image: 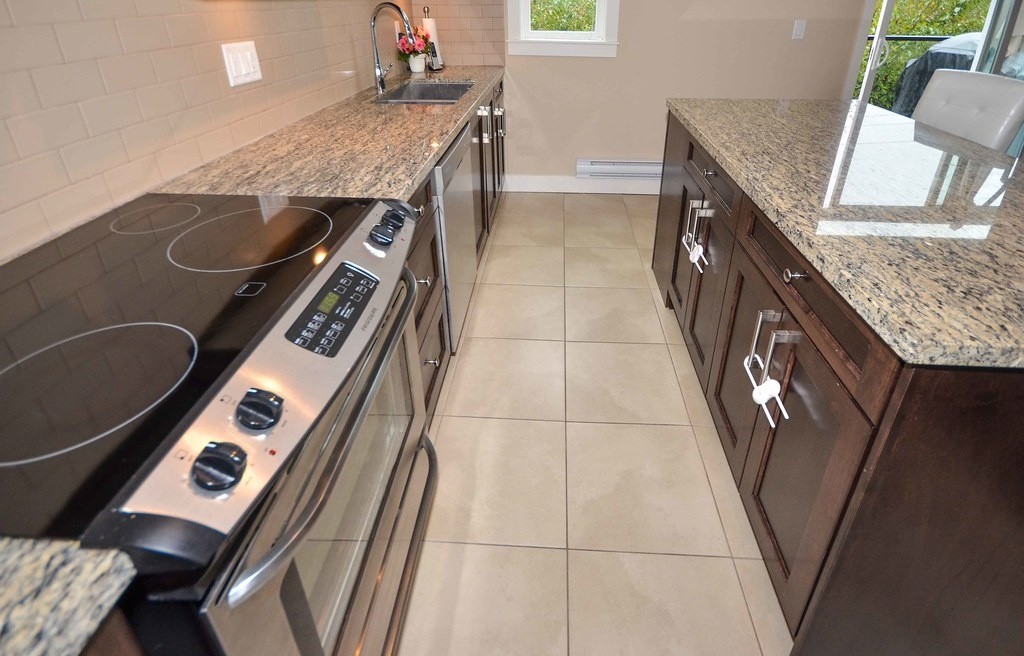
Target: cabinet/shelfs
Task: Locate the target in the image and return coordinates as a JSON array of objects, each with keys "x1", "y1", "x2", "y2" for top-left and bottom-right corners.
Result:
[
  {"x1": 651, "y1": 111, "x2": 1024, "y2": 656},
  {"x1": 469, "y1": 76, "x2": 506, "y2": 269},
  {"x1": 407, "y1": 167, "x2": 452, "y2": 430}
]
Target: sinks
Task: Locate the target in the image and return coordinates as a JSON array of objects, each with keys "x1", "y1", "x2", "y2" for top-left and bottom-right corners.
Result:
[{"x1": 372, "y1": 81, "x2": 477, "y2": 104}]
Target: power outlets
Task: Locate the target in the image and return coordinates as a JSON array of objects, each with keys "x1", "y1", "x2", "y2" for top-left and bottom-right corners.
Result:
[
  {"x1": 792, "y1": 20, "x2": 806, "y2": 39},
  {"x1": 394, "y1": 20, "x2": 400, "y2": 43}
]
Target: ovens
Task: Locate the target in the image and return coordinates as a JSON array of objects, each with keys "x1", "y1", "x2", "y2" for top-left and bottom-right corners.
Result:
[{"x1": 78, "y1": 263, "x2": 438, "y2": 656}]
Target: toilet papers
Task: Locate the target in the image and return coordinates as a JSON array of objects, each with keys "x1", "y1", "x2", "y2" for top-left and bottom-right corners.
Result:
[{"x1": 422, "y1": 17, "x2": 444, "y2": 65}]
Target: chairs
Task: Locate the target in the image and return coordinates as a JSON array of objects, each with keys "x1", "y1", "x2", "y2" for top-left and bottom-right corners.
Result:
[{"x1": 911, "y1": 67, "x2": 1024, "y2": 154}]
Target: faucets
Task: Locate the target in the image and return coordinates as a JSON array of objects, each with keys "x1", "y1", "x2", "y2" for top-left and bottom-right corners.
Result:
[{"x1": 369, "y1": 1, "x2": 416, "y2": 91}]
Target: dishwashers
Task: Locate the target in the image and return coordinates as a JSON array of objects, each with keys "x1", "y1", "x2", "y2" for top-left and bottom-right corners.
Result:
[{"x1": 429, "y1": 121, "x2": 478, "y2": 354}]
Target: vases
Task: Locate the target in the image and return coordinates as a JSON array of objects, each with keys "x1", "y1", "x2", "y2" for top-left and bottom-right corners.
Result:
[{"x1": 408, "y1": 54, "x2": 426, "y2": 73}]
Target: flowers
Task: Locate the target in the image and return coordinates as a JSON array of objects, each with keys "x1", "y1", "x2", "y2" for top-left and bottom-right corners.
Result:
[{"x1": 396, "y1": 24, "x2": 433, "y2": 64}]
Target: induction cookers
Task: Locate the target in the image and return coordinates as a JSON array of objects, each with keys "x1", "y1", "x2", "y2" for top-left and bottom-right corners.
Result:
[{"x1": 0, "y1": 192, "x2": 421, "y2": 570}]
